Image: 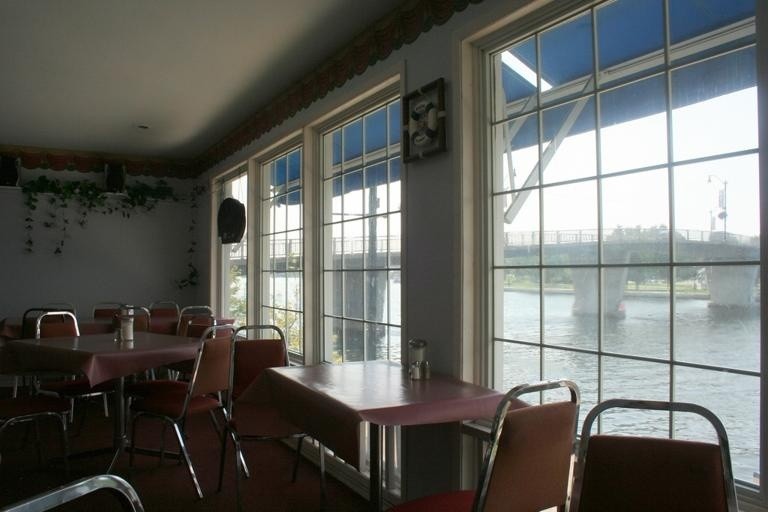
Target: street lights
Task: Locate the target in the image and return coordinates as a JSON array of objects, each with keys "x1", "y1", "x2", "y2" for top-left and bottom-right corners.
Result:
[{"x1": 707, "y1": 172, "x2": 726, "y2": 244}]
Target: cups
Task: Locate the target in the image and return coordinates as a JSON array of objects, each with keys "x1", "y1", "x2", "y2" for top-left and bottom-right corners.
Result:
[
  {"x1": 407, "y1": 339, "x2": 430, "y2": 381},
  {"x1": 112, "y1": 303, "x2": 135, "y2": 342}
]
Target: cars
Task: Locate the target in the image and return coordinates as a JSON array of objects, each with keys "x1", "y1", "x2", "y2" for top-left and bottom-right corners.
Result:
[
  {"x1": 653, "y1": 231, "x2": 684, "y2": 241},
  {"x1": 707, "y1": 230, "x2": 738, "y2": 245}
]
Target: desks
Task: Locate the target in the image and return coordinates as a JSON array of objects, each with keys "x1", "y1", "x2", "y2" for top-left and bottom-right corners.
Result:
[{"x1": 263, "y1": 361, "x2": 534, "y2": 510}]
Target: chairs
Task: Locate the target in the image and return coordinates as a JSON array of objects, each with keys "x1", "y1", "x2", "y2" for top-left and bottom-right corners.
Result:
[
  {"x1": 216, "y1": 324, "x2": 326, "y2": 510},
  {"x1": 1, "y1": 296, "x2": 249, "y2": 512},
  {"x1": 569, "y1": 398, "x2": 740, "y2": 510},
  {"x1": 386, "y1": 378, "x2": 580, "y2": 511}
]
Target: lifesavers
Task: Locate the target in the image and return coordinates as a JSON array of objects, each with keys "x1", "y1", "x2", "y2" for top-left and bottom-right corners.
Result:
[{"x1": 409, "y1": 100, "x2": 438, "y2": 147}]
